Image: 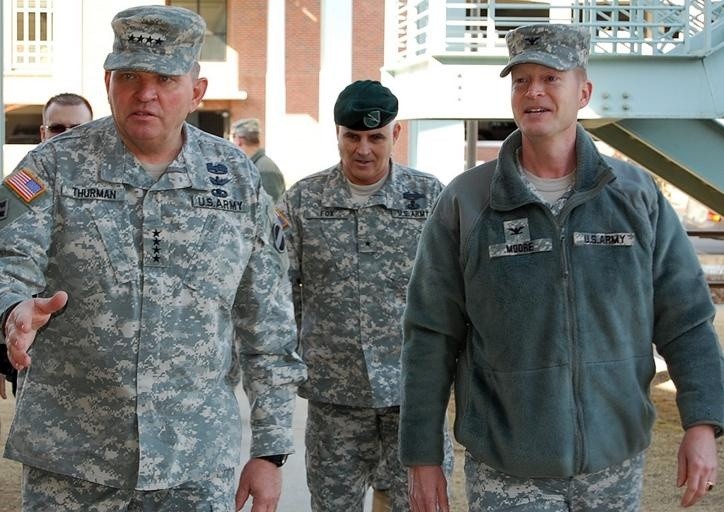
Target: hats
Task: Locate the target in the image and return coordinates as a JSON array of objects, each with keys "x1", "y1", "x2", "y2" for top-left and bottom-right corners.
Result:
[
  {"x1": 500, "y1": 24, "x2": 590, "y2": 78},
  {"x1": 230, "y1": 118, "x2": 260, "y2": 134},
  {"x1": 334, "y1": 80, "x2": 401, "y2": 130},
  {"x1": 104, "y1": 5, "x2": 206, "y2": 76}
]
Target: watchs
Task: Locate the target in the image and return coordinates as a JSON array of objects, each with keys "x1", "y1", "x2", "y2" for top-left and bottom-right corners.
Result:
[{"x1": 256, "y1": 454, "x2": 288, "y2": 468}]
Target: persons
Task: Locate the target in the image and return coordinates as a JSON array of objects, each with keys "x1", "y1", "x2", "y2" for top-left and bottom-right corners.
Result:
[
  {"x1": 395, "y1": 23, "x2": 724, "y2": 512},
  {"x1": 274, "y1": 79, "x2": 455, "y2": 512},
  {"x1": 0, "y1": 3, "x2": 309, "y2": 512},
  {"x1": 228, "y1": 117, "x2": 287, "y2": 208},
  {"x1": 0, "y1": 93, "x2": 93, "y2": 402}
]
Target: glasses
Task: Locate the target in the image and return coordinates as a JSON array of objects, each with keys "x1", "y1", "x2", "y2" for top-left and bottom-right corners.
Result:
[{"x1": 43, "y1": 124, "x2": 80, "y2": 132}]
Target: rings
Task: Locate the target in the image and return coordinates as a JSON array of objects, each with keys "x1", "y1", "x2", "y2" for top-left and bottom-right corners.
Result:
[{"x1": 706, "y1": 481, "x2": 714, "y2": 491}]
